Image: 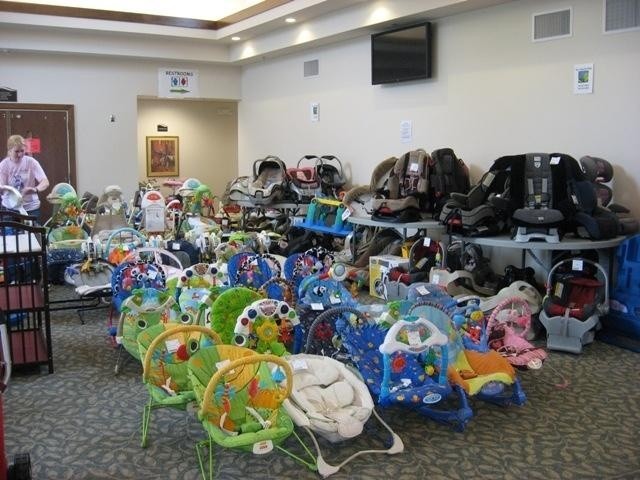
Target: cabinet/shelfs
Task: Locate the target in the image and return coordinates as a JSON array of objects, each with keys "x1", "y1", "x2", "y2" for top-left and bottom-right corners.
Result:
[{"x1": 1, "y1": 210, "x2": 55, "y2": 374}]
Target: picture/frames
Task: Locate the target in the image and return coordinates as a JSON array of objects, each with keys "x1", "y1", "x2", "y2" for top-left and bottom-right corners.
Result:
[{"x1": 146, "y1": 136, "x2": 179, "y2": 177}]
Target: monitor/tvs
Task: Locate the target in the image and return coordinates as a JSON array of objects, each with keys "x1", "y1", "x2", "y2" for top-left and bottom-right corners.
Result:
[{"x1": 371, "y1": 21, "x2": 431, "y2": 86}]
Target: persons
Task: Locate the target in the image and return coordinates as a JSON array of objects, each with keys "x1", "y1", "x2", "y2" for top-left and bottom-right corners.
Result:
[{"x1": 0, "y1": 134, "x2": 50, "y2": 223}]
